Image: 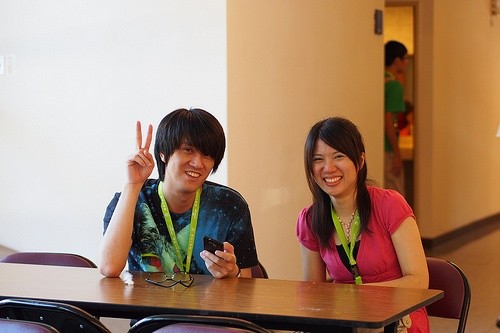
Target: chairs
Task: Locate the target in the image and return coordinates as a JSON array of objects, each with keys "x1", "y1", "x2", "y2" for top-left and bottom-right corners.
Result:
[{"x1": 0, "y1": 253, "x2": 472, "y2": 333}]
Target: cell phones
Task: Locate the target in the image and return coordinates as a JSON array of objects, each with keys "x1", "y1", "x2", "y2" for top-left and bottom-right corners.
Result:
[{"x1": 203, "y1": 236, "x2": 224, "y2": 255}]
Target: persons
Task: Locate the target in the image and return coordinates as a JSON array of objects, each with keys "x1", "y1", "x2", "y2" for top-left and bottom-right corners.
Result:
[
  {"x1": 295, "y1": 117, "x2": 430, "y2": 333},
  {"x1": 384, "y1": 40, "x2": 409, "y2": 201},
  {"x1": 97, "y1": 108, "x2": 259, "y2": 328}
]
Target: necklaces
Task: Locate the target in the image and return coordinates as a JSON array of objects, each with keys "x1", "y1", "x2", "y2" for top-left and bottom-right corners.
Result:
[{"x1": 337, "y1": 207, "x2": 358, "y2": 241}]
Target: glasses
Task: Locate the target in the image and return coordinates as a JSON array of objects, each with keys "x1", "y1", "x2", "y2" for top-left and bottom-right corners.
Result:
[{"x1": 145, "y1": 271, "x2": 194, "y2": 288}]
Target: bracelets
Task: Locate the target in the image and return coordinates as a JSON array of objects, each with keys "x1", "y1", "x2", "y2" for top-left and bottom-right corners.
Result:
[{"x1": 236, "y1": 263, "x2": 241, "y2": 277}]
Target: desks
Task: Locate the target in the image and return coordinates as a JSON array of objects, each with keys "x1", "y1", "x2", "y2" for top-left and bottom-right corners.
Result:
[{"x1": 0, "y1": 261, "x2": 444, "y2": 333}]
